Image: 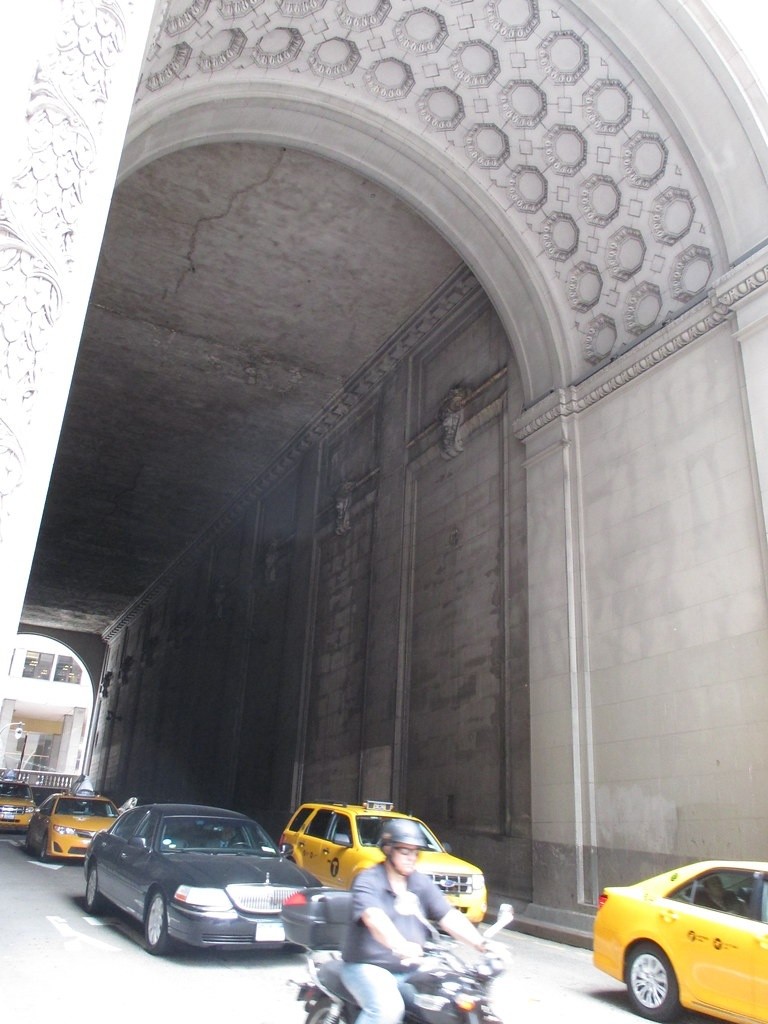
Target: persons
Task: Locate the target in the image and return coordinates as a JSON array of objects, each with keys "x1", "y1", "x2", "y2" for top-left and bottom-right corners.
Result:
[
  {"x1": 339, "y1": 819, "x2": 497, "y2": 1024},
  {"x1": 704, "y1": 875, "x2": 745, "y2": 917},
  {"x1": 205, "y1": 823, "x2": 245, "y2": 854}
]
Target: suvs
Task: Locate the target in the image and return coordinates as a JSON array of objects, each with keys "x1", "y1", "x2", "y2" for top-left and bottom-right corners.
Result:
[{"x1": 278, "y1": 799, "x2": 488, "y2": 929}]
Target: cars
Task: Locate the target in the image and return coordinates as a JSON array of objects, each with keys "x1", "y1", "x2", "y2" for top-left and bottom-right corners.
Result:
[
  {"x1": 28, "y1": 775, "x2": 120, "y2": 866},
  {"x1": 590, "y1": 859, "x2": 768, "y2": 1024},
  {"x1": 83, "y1": 802, "x2": 324, "y2": 958},
  {"x1": 1, "y1": 769, "x2": 38, "y2": 835}
]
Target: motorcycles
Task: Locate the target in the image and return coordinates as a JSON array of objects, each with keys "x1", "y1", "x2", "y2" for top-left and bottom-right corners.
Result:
[{"x1": 279, "y1": 887, "x2": 516, "y2": 1024}]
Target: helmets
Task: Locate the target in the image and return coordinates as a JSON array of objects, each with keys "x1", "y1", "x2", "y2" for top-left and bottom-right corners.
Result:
[{"x1": 380, "y1": 818, "x2": 428, "y2": 846}]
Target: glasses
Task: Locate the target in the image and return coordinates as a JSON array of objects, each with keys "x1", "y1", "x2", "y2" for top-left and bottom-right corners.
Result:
[{"x1": 393, "y1": 847, "x2": 419, "y2": 856}]
